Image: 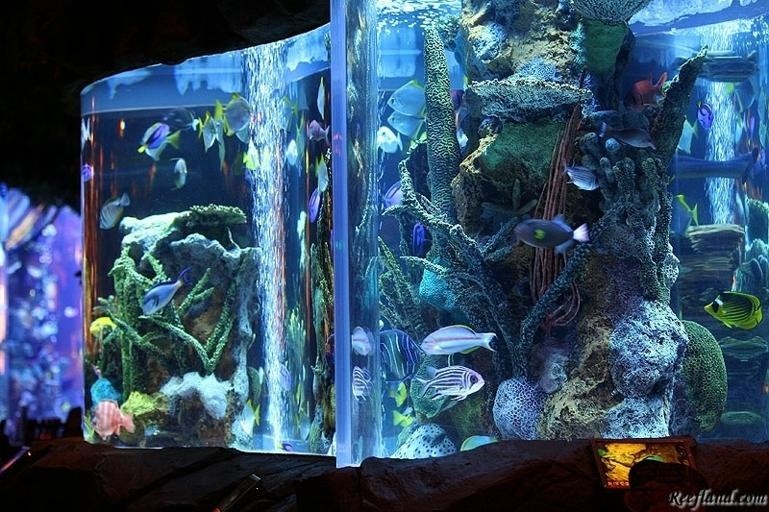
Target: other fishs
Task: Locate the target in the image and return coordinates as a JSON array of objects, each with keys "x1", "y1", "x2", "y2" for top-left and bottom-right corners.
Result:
[{"x1": 284, "y1": 76, "x2": 499, "y2": 453}]
